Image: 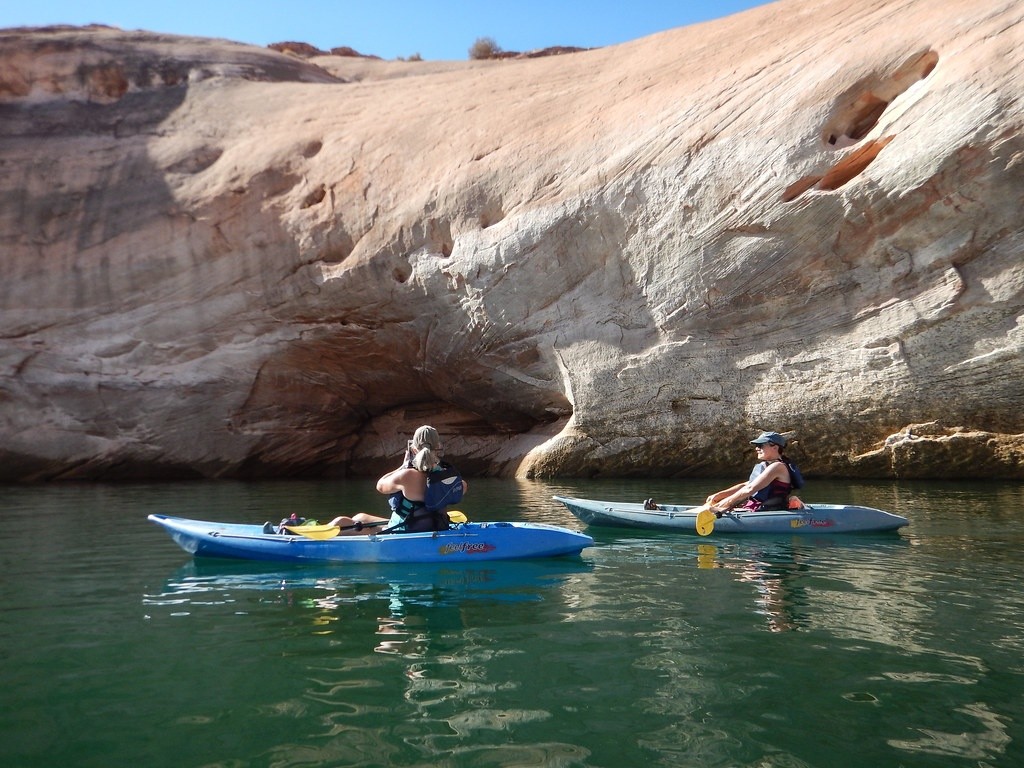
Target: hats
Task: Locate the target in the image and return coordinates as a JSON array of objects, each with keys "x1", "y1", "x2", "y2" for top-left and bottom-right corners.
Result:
[
  {"x1": 749, "y1": 432, "x2": 786, "y2": 447},
  {"x1": 413, "y1": 425, "x2": 441, "y2": 452}
]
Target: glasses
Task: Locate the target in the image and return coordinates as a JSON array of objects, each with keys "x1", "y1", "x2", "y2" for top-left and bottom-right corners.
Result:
[{"x1": 756, "y1": 444, "x2": 764, "y2": 448}]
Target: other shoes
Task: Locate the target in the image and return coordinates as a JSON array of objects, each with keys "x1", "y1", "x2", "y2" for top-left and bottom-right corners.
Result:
[
  {"x1": 643, "y1": 500, "x2": 651, "y2": 510},
  {"x1": 262, "y1": 521, "x2": 276, "y2": 534},
  {"x1": 649, "y1": 498, "x2": 658, "y2": 510}
]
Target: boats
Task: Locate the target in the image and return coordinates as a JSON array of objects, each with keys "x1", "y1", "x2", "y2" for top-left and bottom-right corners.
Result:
[
  {"x1": 552, "y1": 495, "x2": 910, "y2": 535},
  {"x1": 149, "y1": 513, "x2": 595, "y2": 562}
]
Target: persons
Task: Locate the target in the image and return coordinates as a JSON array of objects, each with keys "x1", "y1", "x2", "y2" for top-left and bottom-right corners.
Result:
[
  {"x1": 262, "y1": 425, "x2": 467, "y2": 537},
  {"x1": 644, "y1": 432, "x2": 791, "y2": 514}
]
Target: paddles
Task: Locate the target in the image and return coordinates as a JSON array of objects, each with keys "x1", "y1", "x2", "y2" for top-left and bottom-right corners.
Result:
[
  {"x1": 695, "y1": 505, "x2": 738, "y2": 536},
  {"x1": 282, "y1": 510, "x2": 468, "y2": 540}
]
